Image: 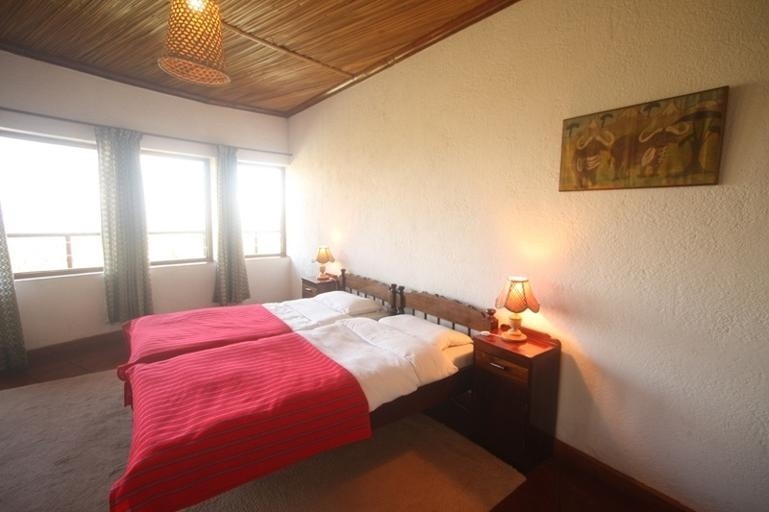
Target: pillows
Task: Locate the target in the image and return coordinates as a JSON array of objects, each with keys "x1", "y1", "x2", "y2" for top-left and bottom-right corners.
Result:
[
  {"x1": 377, "y1": 311, "x2": 475, "y2": 352},
  {"x1": 314, "y1": 290, "x2": 384, "y2": 315}
]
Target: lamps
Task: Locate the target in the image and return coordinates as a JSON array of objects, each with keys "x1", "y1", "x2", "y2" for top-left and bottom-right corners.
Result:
[
  {"x1": 311, "y1": 244, "x2": 336, "y2": 281},
  {"x1": 494, "y1": 276, "x2": 542, "y2": 343},
  {"x1": 157, "y1": 1, "x2": 232, "y2": 87}
]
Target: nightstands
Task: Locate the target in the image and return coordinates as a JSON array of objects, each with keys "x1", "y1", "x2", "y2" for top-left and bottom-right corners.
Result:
[
  {"x1": 301, "y1": 273, "x2": 338, "y2": 298},
  {"x1": 466, "y1": 323, "x2": 562, "y2": 476}
]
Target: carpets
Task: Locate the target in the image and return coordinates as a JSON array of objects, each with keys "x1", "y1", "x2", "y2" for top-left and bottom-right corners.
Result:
[{"x1": 1, "y1": 366, "x2": 527, "y2": 512}]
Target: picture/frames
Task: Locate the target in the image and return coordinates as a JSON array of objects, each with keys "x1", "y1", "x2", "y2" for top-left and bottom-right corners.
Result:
[{"x1": 557, "y1": 85, "x2": 730, "y2": 194}]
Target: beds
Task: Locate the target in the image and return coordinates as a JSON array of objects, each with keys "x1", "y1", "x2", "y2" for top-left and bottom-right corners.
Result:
[
  {"x1": 108, "y1": 285, "x2": 500, "y2": 512},
  {"x1": 115, "y1": 269, "x2": 398, "y2": 410}
]
